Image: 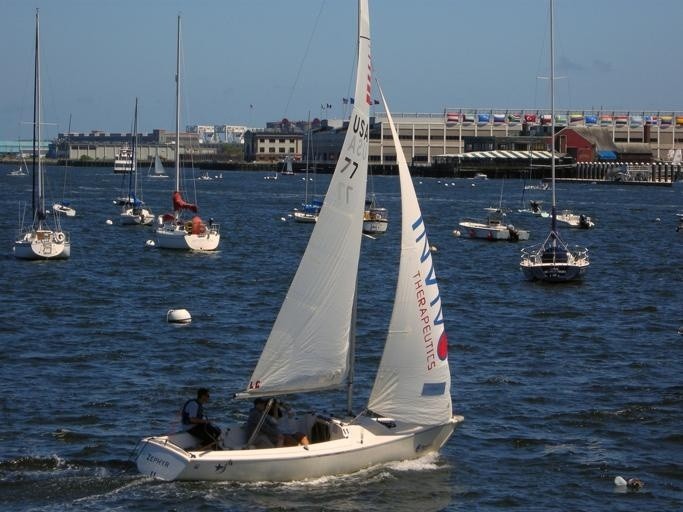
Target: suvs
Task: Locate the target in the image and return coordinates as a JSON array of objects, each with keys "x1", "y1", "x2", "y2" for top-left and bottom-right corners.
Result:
[{"x1": 615, "y1": 172, "x2": 632, "y2": 181}]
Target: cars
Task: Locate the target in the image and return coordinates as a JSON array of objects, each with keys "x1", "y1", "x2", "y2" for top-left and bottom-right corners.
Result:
[{"x1": 634, "y1": 172, "x2": 650, "y2": 181}]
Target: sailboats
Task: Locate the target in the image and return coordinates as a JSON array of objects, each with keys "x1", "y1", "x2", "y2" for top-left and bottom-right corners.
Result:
[
  {"x1": 519, "y1": 0, "x2": 593, "y2": 287},
  {"x1": 6, "y1": 9, "x2": 76, "y2": 264},
  {"x1": 130, "y1": 2, "x2": 465, "y2": 491},
  {"x1": 517, "y1": 148, "x2": 543, "y2": 215},
  {"x1": 281, "y1": 111, "x2": 393, "y2": 240},
  {"x1": 149, "y1": 12, "x2": 225, "y2": 256},
  {"x1": 113, "y1": 98, "x2": 171, "y2": 231}
]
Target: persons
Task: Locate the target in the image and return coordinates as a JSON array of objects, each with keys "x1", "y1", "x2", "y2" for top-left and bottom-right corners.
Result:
[
  {"x1": 266, "y1": 398, "x2": 287, "y2": 447},
  {"x1": 179, "y1": 386, "x2": 224, "y2": 452},
  {"x1": 244, "y1": 397, "x2": 277, "y2": 449}
]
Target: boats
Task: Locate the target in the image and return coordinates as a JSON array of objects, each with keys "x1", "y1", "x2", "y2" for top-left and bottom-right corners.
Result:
[
  {"x1": 540, "y1": 211, "x2": 595, "y2": 229},
  {"x1": 458, "y1": 206, "x2": 531, "y2": 243}
]
